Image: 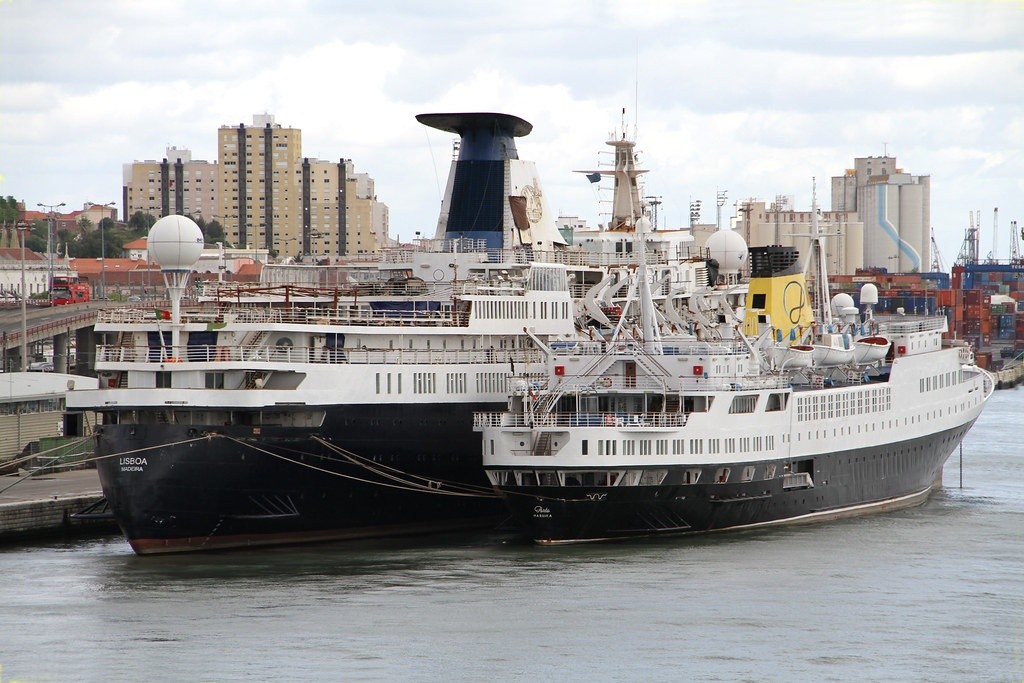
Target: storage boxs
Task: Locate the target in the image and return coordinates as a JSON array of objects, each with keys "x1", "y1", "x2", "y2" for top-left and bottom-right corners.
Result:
[{"x1": 828, "y1": 271, "x2": 1024, "y2": 352}]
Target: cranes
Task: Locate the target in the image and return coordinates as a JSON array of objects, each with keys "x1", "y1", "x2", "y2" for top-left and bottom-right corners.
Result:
[
  {"x1": 955, "y1": 207, "x2": 1021, "y2": 266},
  {"x1": 931, "y1": 228, "x2": 945, "y2": 273}
]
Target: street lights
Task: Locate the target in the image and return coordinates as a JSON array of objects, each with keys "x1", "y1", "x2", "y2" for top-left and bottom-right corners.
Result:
[
  {"x1": 177, "y1": 210, "x2": 202, "y2": 216},
  {"x1": 213, "y1": 214, "x2": 236, "y2": 281},
  {"x1": 88, "y1": 202, "x2": 116, "y2": 299},
  {"x1": 38, "y1": 203, "x2": 66, "y2": 307},
  {"x1": 135, "y1": 208, "x2": 159, "y2": 286},
  {"x1": 275, "y1": 238, "x2": 297, "y2": 265}
]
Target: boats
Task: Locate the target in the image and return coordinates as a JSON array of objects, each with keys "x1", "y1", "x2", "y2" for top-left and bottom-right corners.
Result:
[
  {"x1": 62, "y1": 78, "x2": 755, "y2": 556},
  {"x1": 480, "y1": 176, "x2": 995, "y2": 547},
  {"x1": 768, "y1": 333, "x2": 891, "y2": 368}
]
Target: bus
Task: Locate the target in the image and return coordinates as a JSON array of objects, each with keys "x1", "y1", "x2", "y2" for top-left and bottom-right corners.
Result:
[{"x1": 50, "y1": 275, "x2": 90, "y2": 306}]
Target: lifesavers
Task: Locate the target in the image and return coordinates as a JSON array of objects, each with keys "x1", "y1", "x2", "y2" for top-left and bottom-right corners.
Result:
[
  {"x1": 606, "y1": 414, "x2": 614, "y2": 426},
  {"x1": 602, "y1": 377, "x2": 613, "y2": 387}
]
